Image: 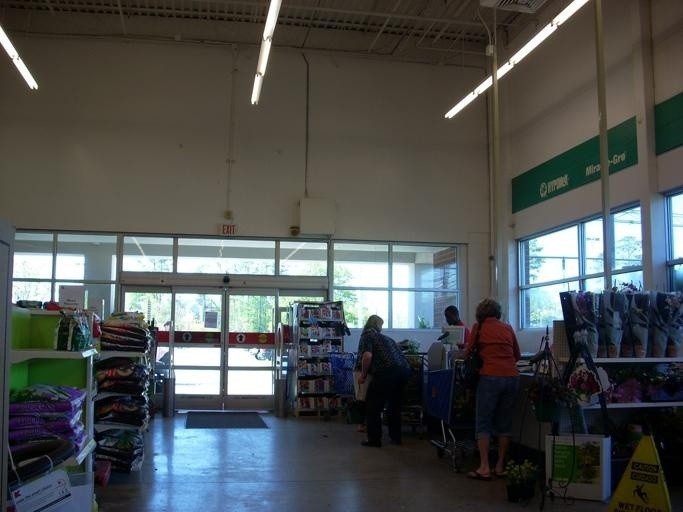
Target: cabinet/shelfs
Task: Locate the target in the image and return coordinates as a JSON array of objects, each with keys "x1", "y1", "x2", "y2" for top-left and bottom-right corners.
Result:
[
  {"x1": 4, "y1": 305, "x2": 99, "y2": 512},
  {"x1": 289, "y1": 300, "x2": 349, "y2": 420},
  {"x1": 551, "y1": 319, "x2": 683, "y2": 469},
  {"x1": 85, "y1": 345, "x2": 149, "y2": 474}
]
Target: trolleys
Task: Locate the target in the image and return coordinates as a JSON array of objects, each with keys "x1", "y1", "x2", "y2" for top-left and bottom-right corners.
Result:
[
  {"x1": 380, "y1": 351, "x2": 428, "y2": 440},
  {"x1": 425, "y1": 358, "x2": 499, "y2": 473},
  {"x1": 322, "y1": 351, "x2": 359, "y2": 424}
]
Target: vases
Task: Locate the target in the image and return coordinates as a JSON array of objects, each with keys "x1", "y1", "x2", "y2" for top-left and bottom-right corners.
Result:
[{"x1": 505, "y1": 481, "x2": 533, "y2": 504}]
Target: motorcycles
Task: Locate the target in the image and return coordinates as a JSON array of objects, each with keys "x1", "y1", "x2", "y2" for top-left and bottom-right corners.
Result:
[{"x1": 245, "y1": 348, "x2": 272, "y2": 361}]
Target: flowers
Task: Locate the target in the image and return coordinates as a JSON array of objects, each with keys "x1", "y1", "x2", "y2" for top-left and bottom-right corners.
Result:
[
  {"x1": 503, "y1": 457, "x2": 536, "y2": 483},
  {"x1": 521, "y1": 279, "x2": 683, "y2": 424}
]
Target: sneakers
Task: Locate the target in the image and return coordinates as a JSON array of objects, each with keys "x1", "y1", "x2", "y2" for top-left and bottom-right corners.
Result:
[
  {"x1": 360, "y1": 432, "x2": 383, "y2": 447},
  {"x1": 389, "y1": 439, "x2": 400, "y2": 446}
]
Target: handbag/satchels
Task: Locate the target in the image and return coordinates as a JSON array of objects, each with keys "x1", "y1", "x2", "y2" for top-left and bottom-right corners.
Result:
[
  {"x1": 543, "y1": 344, "x2": 615, "y2": 503},
  {"x1": 463, "y1": 318, "x2": 483, "y2": 371},
  {"x1": 532, "y1": 346, "x2": 562, "y2": 424},
  {"x1": 344, "y1": 383, "x2": 368, "y2": 424}
]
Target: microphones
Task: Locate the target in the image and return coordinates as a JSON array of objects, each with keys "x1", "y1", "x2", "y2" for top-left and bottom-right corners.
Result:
[{"x1": 437, "y1": 331, "x2": 449, "y2": 340}]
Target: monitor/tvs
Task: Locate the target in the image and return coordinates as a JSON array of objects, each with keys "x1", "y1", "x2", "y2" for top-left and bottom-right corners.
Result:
[{"x1": 440, "y1": 325, "x2": 465, "y2": 345}]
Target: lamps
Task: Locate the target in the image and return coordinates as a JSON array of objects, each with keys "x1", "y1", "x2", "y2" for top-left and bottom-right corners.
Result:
[
  {"x1": 0, "y1": 25, "x2": 37, "y2": 90},
  {"x1": 442, "y1": 0, "x2": 591, "y2": 121},
  {"x1": 247, "y1": 0, "x2": 281, "y2": 106}
]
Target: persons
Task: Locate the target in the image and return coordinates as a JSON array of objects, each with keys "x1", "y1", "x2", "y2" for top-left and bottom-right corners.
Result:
[
  {"x1": 352, "y1": 314, "x2": 384, "y2": 433},
  {"x1": 462, "y1": 297, "x2": 521, "y2": 481},
  {"x1": 444, "y1": 304, "x2": 472, "y2": 351},
  {"x1": 359, "y1": 326, "x2": 412, "y2": 448}
]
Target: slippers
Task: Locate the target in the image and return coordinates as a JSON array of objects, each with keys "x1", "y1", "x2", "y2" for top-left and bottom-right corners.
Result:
[{"x1": 465, "y1": 463, "x2": 508, "y2": 482}]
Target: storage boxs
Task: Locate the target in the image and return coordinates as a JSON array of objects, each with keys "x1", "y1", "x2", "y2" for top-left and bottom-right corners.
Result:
[{"x1": 545, "y1": 432, "x2": 612, "y2": 500}]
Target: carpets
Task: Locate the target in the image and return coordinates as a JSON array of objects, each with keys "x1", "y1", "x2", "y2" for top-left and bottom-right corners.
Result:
[{"x1": 185, "y1": 409, "x2": 268, "y2": 431}]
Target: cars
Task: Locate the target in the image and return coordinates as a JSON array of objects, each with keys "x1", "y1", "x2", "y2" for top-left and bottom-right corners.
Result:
[{"x1": 154, "y1": 347, "x2": 175, "y2": 393}]
